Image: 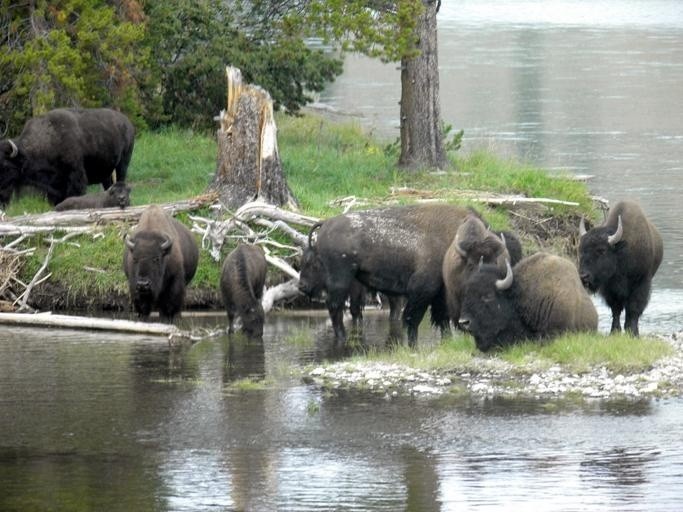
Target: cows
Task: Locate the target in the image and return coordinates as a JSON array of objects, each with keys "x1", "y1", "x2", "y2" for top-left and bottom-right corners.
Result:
[
  {"x1": 576, "y1": 200, "x2": 664, "y2": 340},
  {"x1": 0, "y1": 104, "x2": 135, "y2": 207},
  {"x1": 55, "y1": 182, "x2": 133, "y2": 211},
  {"x1": 218, "y1": 243, "x2": 267, "y2": 339},
  {"x1": 122, "y1": 204, "x2": 199, "y2": 324},
  {"x1": 296, "y1": 199, "x2": 522, "y2": 350},
  {"x1": 459, "y1": 251, "x2": 600, "y2": 353}
]
